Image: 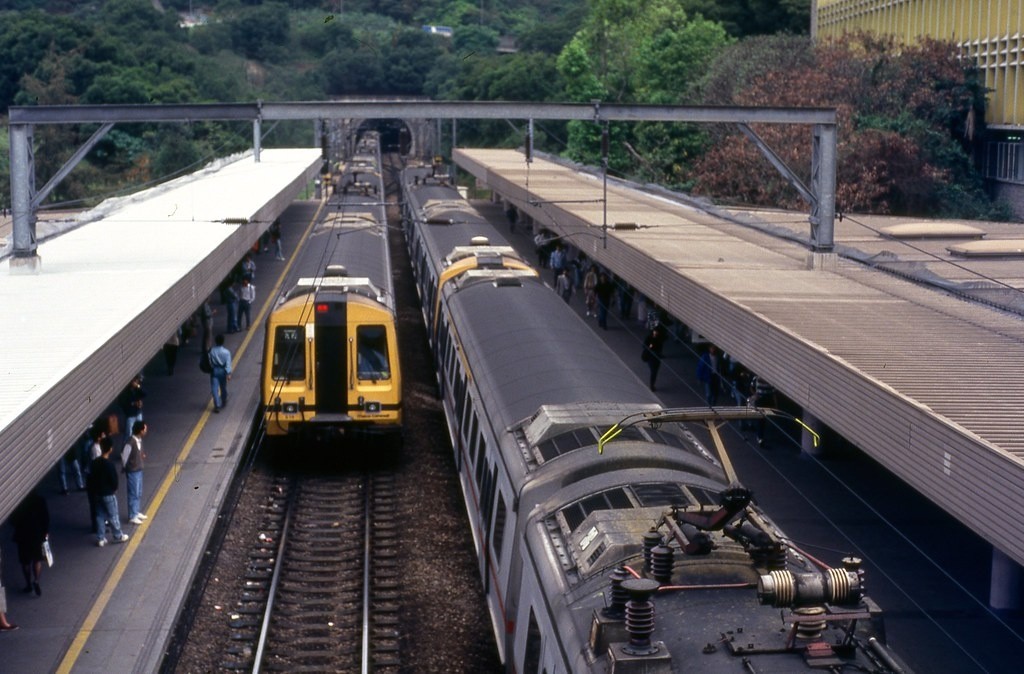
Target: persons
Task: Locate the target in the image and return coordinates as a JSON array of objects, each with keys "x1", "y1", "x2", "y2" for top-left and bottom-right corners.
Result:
[
  {"x1": 11, "y1": 491, "x2": 51, "y2": 597},
  {"x1": 507, "y1": 204, "x2": 518, "y2": 232},
  {"x1": 0, "y1": 579, "x2": 19, "y2": 631},
  {"x1": 156, "y1": 215, "x2": 284, "y2": 413},
  {"x1": 59, "y1": 374, "x2": 148, "y2": 547},
  {"x1": 534, "y1": 220, "x2": 772, "y2": 445}
]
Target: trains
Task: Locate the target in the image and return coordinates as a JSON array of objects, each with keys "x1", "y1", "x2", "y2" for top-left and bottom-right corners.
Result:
[
  {"x1": 396, "y1": 162, "x2": 915, "y2": 674},
  {"x1": 258, "y1": 129, "x2": 406, "y2": 443}
]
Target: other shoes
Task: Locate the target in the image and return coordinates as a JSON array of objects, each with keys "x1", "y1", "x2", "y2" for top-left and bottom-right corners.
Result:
[
  {"x1": 97, "y1": 537, "x2": 108, "y2": 546},
  {"x1": 214, "y1": 407, "x2": 221, "y2": 413},
  {"x1": 129, "y1": 512, "x2": 148, "y2": 524},
  {"x1": 33, "y1": 582, "x2": 42, "y2": 597},
  {"x1": 111, "y1": 533, "x2": 129, "y2": 542},
  {"x1": 586, "y1": 310, "x2": 590, "y2": 316},
  {"x1": 1, "y1": 624, "x2": 20, "y2": 631}
]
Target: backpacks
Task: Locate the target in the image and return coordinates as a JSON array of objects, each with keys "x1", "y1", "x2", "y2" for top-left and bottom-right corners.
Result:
[{"x1": 200, "y1": 349, "x2": 214, "y2": 374}]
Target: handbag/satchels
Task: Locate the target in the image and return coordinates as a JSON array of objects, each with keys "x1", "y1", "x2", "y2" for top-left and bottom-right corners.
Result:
[{"x1": 41, "y1": 540, "x2": 54, "y2": 568}]
